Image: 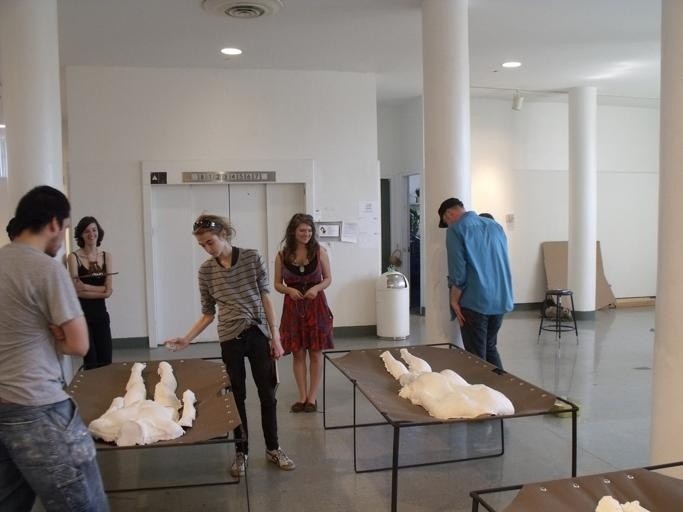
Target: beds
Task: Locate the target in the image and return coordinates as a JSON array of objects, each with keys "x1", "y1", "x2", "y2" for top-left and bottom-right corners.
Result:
[
  {"x1": 470, "y1": 459, "x2": 682, "y2": 512},
  {"x1": 63, "y1": 355, "x2": 248, "y2": 511},
  {"x1": 321, "y1": 340, "x2": 578, "y2": 512}
]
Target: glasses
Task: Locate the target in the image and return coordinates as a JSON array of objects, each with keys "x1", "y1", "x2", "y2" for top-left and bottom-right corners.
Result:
[{"x1": 193, "y1": 220, "x2": 221, "y2": 230}]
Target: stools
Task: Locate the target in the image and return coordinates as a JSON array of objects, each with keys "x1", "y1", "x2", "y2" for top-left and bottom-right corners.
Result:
[{"x1": 536, "y1": 289, "x2": 579, "y2": 339}]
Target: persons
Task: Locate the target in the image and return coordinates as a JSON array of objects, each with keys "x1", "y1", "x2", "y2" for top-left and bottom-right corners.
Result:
[
  {"x1": 439, "y1": 197, "x2": 515, "y2": 371},
  {"x1": 274, "y1": 213, "x2": 336, "y2": 412},
  {"x1": 4, "y1": 217, "x2": 16, "y2": 239},
  {"x1": 164, "y1": 212, "x2": 297, "y2": 479},
  {"x1": 1, "y1": 185, "x2": 116, "y2": 512},
  {"x1": 65, "y1": 218, "x2": 116, "y2": 368}
]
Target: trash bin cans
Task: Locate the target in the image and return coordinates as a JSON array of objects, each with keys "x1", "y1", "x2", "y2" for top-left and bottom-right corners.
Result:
[{"x1": 375, "y1": 271, "x2": 410, "y2": 342}]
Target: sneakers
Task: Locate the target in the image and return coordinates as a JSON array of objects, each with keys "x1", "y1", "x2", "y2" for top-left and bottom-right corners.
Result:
[
  {"x1": 265, "y1": 447, "x2": 295, "y2": 470},
  {"x1": 231, "y1": 454, "x2": 247, "y2": 476},
  {"x1": 304, "y1": 398, "x2": 316, "y2": 412},
  {"x1": 292, "y1": 402, "x2": 304, "y2": 413}
]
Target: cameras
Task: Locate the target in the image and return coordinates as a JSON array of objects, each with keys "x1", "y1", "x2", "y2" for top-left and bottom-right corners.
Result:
[{"x1": 165, "y1": 342, "x2": 177, "y2": 352}]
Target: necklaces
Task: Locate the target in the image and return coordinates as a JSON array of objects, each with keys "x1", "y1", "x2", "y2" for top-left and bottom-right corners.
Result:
[{"x1": 291, "y1": 253, "x2": 312, "y2": 276}]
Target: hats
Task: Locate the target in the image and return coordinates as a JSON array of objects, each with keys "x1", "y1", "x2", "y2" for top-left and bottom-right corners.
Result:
[{"x1": 438, "y1": 198, "x2": 463, "y2": 228}]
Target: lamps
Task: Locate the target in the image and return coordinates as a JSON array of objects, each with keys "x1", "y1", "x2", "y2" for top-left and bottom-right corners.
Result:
[{"x1": 510, "y1": 89, "x2": 527, "y2": 111}]
